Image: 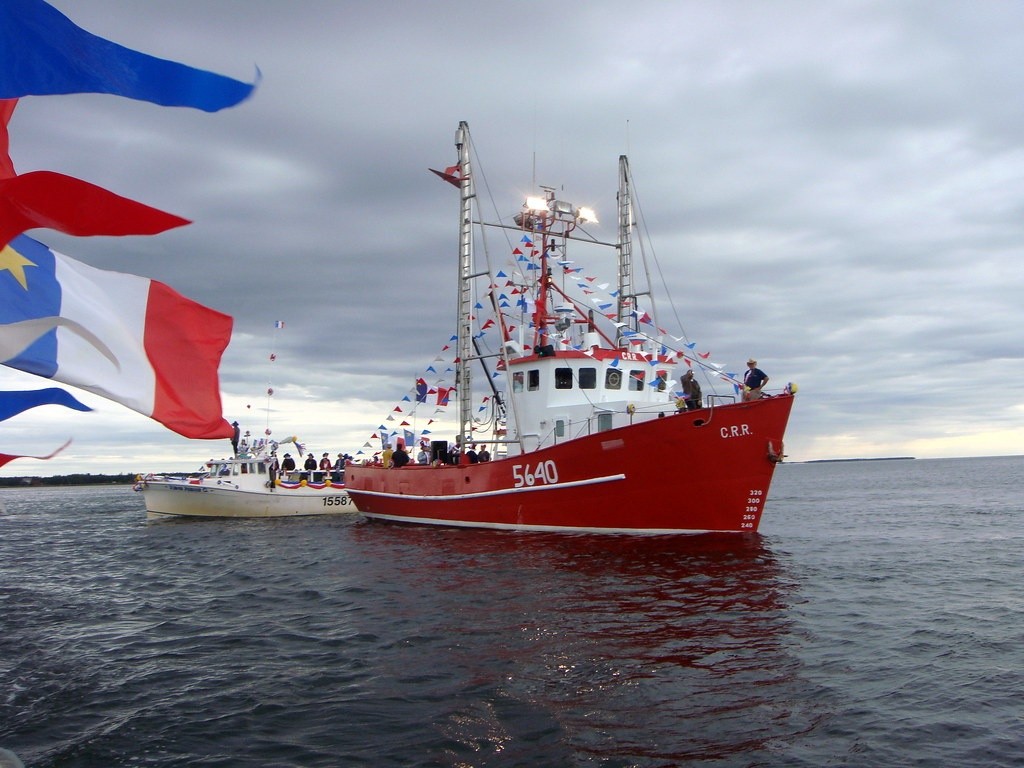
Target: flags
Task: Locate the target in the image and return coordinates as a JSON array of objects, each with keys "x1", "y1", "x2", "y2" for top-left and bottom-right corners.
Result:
[
  {"x1": 0, "y1": 234, "x2": 235, "y2": 440},
  {"x1": 416, "y1": 379, "x2": 449, "y2": 406},
  {"x1": 381, "y1": 432, "x2": 405, "y2": 450},
  {"x1": 275, "y1": 321, "x2": 285, "y2": 329},
  {"x1": 404, "y1": 430, "x2": 431, "y2": 446}
]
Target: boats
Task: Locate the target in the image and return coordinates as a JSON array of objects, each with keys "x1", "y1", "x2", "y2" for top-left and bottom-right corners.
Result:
[
  {"x1": 132, "y1": 438, "x2": 369, "y2": 520},
  {"x1": 344, "y1": 118, "x2": 797, "y2": 536}
]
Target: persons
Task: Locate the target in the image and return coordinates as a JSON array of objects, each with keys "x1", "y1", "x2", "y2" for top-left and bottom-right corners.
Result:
[
  {"x1": 282, "y1": 453, "x2": 295, "y2": 470},
  {"x1": 230, "y1": 422, "x2": 240, "y2": 453},
  {"x1": 304, "y1": 453, "x2": 317, "y2": 470},
  {"x1": 382, "y1": 444, "x2": 393, "y2": 468},
  {"x1": 448, "y1": 444, "x2": 489, "y2": 465},
  {"x1": 743, "y1": 359, "x2": 769, "y2": 401},
  {"x1": 417, "y1": 447, "x2": 427, "y2": 464},
  {"x1": 320, "y1": 453, "x2": 331, "y2": 470},
  {"x1": 334, "y1": 453, "x2": 345, "y2": 468},
  {"x1": 371, "y1": 456, "x2": 380, "y2": 464},
  {"x1": 392, "y1": 443, "x2": 410, "y2": 467},
  {"x1": 680, "y1": 369, "x2": 703, "y2": 410},
  {"x1": 270, "y1": 451, "x2": 279, "y2": 470}
]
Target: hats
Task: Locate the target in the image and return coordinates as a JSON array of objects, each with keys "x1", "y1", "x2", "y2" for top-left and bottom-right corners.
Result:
[
  {"x1": 307, "y1": 453, "x2": 314, "y2": 458},
  {"x1": 283, "y1": 453, "x2": 290, "y2": 457},
  {"x1": 322, "y1": 452, "x2": 329, "y2": 457},
  {"x1": 746, "y1": 358, "x2": 757, "y2": 367},
  {"x1": 338, "y1": 453, "x2": 343, "y2": 456},
  {"x1": 232, "y1": 421, "x2": 239, "y2": 426}
]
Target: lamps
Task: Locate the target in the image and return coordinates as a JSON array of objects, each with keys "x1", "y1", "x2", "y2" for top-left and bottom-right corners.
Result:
[
  {"x1": 555, "y1": 317, "x2": 570, "y2": 338},
  {"x1": 577, "y1": 207, "x2": 599, "y2": 223},
  {"x1": 522, "y1": 197, "x2": 549, "y2": 210}
]
